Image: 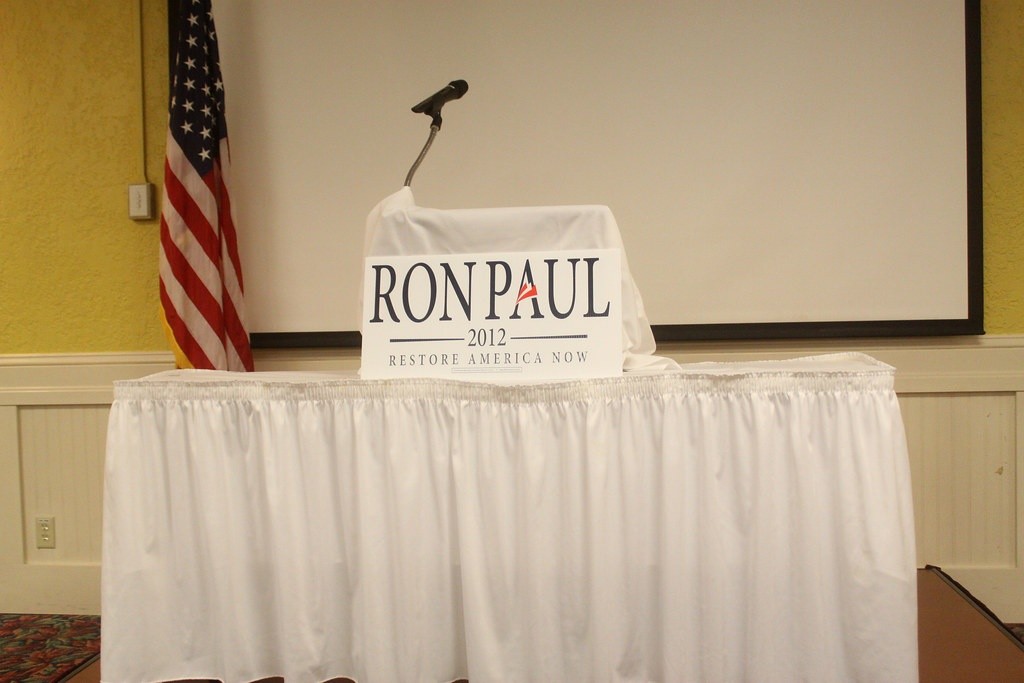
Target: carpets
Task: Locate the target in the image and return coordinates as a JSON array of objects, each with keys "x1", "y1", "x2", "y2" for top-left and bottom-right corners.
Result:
[{"x1": 0, "y1": 612, "x2": 100, "y2": 683}]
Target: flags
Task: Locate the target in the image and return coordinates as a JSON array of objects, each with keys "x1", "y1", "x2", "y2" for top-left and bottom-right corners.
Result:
[{"x1": 158, "y1": 0, "x2": 254, "y2": 374}]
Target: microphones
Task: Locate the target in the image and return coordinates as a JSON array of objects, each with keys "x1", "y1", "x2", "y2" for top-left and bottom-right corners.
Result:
[{"x1": 412, "y1": 80, "x2": 469, "y2": 113}]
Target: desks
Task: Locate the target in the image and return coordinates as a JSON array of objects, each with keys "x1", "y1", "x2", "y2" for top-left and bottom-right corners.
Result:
[{"x1": 98, "y1": 350, "x2": 923, "y2": 683}]
